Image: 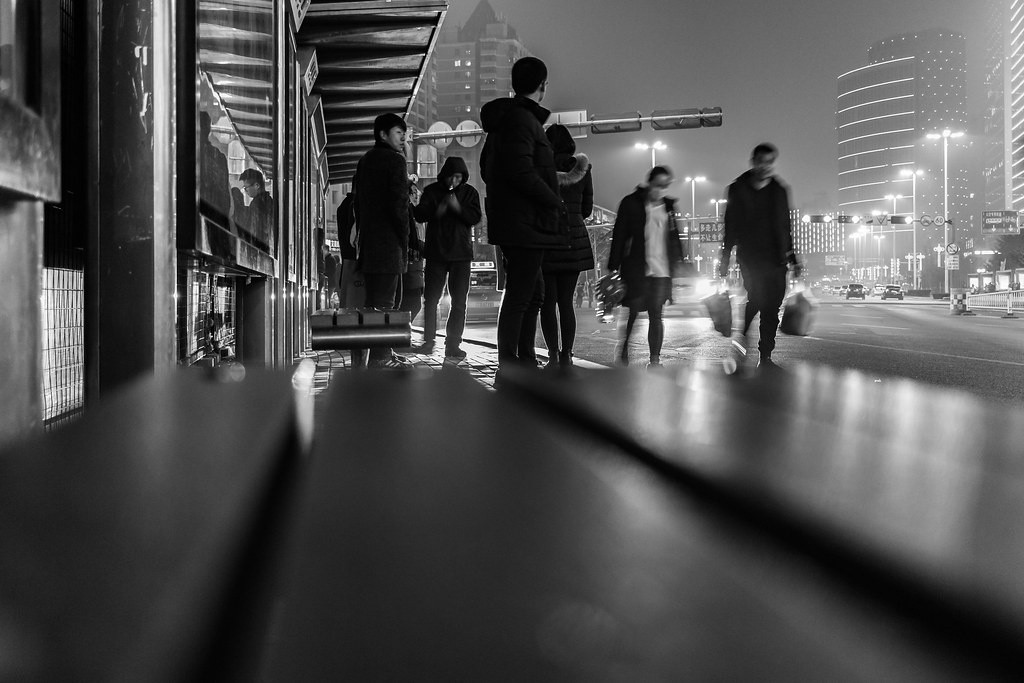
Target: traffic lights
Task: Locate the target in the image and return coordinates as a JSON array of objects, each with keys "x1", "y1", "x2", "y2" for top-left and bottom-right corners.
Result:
[
  {"x1": 891, "y1": 216, "x2": 913, "y2": 224},
  {"x1": 802, "y1": 215, "x2": 831, "y2": 223},
  {"x1": 838, "y1": 215, "x2": 860, "y2": 223}
]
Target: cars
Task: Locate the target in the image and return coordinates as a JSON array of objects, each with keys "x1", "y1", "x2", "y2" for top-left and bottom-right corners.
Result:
[
  {"x1": 880, "y1": 286, "x2": 903, "y2": 300},
  {"x1": 441, "y1": 261, "x2": 503, "y2": 321},
  {"x1": 638, "y1": 260, "x2": 728, "y2": 319},
  {"x1": 839, "y1": 286, "x2": 848, "y2": 296},
  {"x1": 863, "y1": 285, "x2": 885, "y2": 297},
  {"x1": 822, "y1": 285, "x2": 841, "y2": 295}
]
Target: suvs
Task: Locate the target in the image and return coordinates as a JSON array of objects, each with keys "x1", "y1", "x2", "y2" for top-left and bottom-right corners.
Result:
[{"x1": 846, "y1": 284, "x2": 865, "y2": 300}]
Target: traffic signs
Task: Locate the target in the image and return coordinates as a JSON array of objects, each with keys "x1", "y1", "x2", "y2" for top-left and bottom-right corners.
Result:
[{"x1": 981, "y1": 210, "x2": 1020, "y2": 235}]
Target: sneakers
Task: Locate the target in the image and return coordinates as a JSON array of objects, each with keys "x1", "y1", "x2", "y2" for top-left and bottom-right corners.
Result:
[{"x1": 368, "y1": 348, "x2": 414, "y2": 371}]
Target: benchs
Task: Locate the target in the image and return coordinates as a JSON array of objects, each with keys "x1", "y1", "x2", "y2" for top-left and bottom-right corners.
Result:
[{"x1": 308, "y1": 307, "x2": 412, "y2": 369}]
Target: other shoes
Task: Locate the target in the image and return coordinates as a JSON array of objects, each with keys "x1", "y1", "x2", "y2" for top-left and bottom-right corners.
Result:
[
  {"x1": 548, "y1": 349, "x2": 574, "y2": 365},
  {"x1": 757, "y1": 359, "x2": 785, "y2": 380},
  {"x1": 413, "y1": 341, "x2": 436, "y2": 355},
  {"x1": 445, "y1": 345, "x2": 466, "y2": 358}
]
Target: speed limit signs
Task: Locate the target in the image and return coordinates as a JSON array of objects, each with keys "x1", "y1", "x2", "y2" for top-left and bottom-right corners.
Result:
[{"x1": 933, "y1": 215, "x2": 944, "y2": 226}]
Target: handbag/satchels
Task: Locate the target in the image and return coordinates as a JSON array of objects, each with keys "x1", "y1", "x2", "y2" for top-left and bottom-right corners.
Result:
[
  {"x1": 703, "y1": 271, "x2": 733, "y2": 338},
  {"x1": 339, "y1": 241, "x2": 367, "y2": 308},
  {"x1": 780, "y1": 275, "x2": 814, "y2": 336},
  {"x1": 594, "y1": 264, "x2": 624, "y2": 323}
]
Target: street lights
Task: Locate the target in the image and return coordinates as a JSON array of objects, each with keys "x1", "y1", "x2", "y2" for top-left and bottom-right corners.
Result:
[
  {"x1": 850, "y1": 211, "x2": 888, "y2": 285},
  {"x1": 686, "y1": 176, "x2": 705, "y2": 264},
  {"x1": 886, "y1": 193, "x2": 904, "y2": 284},
  {"x1": 711, "y1": 199, "x2": 728, "y2": 220},
  {"x1": 900, "y1": 170, "x2": 925, "y2": 289},
  {"x1": 925, "y1": 129, "x2": 965, "y2": 292},
  {"x1": 635, "y1": 140, "x2": 668, "y2": 169}
]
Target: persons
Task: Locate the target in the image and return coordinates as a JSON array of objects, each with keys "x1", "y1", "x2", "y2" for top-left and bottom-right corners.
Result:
[
  {"x1": 987, "y1": 282, "x2": 993, "y2": 292},
  {"x1": 546, "y1": 124, "x2": 594, "y2": 372},
  {"x1": 199, "y1": 110, "x2": 232, "y2": 260},
  {"x1": 480, "y1": 57, "x2": 555, "y2": 394},
  {"x1": 970, "y1": 284, "x2": 975, "y2": 295},
  {"x1": 316, "y1": 228, "x2": 341, "y2": 309},
  {"x1": 337, "y1": 114, "x2": 425, "y2": 373},
  {"x1": 316, "y1": 243, "x2": 341, "y2": 311},
  {"x1": 414, "y1": 159, "x2": 483, "y2": 357},
  {"x1": 233, "y1": 167, "x2": 277, "y2": 245},
  {"x1": 575, "y1": 279, "x2": 593, "y2": 308},
  {"x1": 1012, "y1": 282, "x2": 1020, "y2": 290},
  {"x1": 608, "y1": 166, "x2": 685, "y2": 373},
  {"x1": 719, "y1": 142, "x2": 802, "y2": 374}
]
